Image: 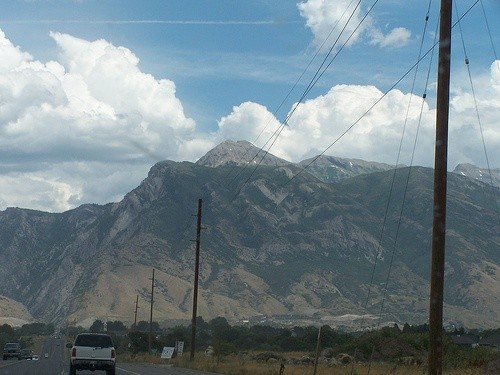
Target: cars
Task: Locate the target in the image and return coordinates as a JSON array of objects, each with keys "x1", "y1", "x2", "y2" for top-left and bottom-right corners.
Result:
[{"x1": 19, "y1": 349, "x2": 32, "y2": 360}]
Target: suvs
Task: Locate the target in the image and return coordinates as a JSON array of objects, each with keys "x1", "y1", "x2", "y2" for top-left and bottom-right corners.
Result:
[{"x1": 2, "y1": 343, "x2": 21, "y2": 360}]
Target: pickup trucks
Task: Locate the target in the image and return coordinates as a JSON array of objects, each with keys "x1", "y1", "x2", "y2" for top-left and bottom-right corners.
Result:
[{"x1": 66, "y1": 333, "x2": 117, "y2": 375}]
